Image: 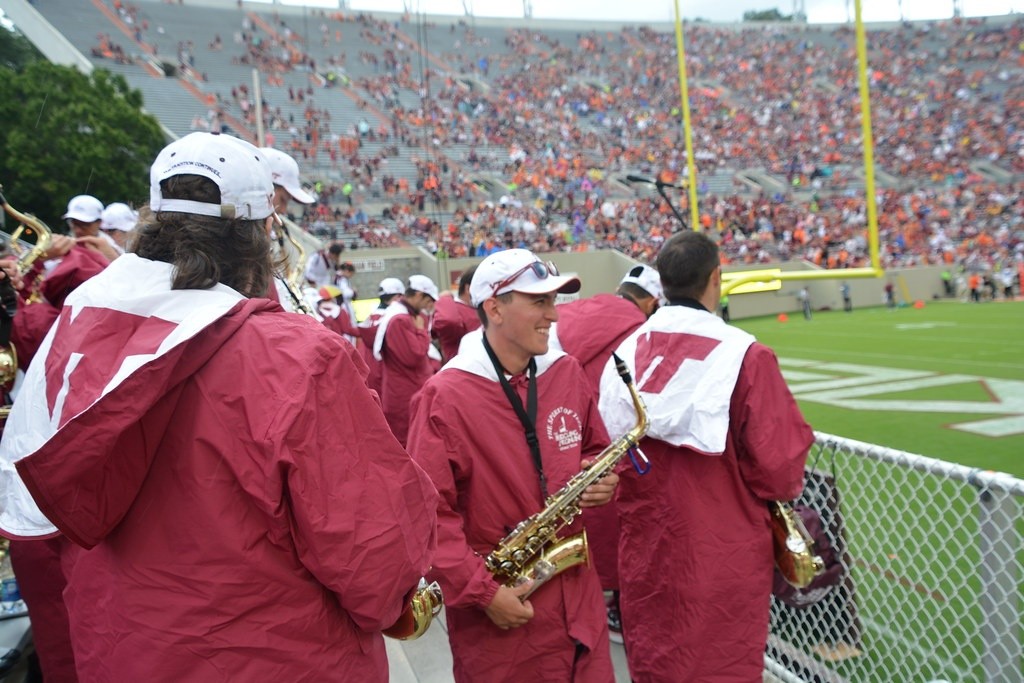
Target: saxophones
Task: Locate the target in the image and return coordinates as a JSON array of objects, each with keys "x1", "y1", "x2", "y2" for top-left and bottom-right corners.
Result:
[
  {"x1": 0, "y1": 186, "x2": 54, "y2": 418},
  {"x1": 387, "y1": 347, "x2": 655, "y2": 643},
  {"x1": 771, "y1": 485, "x2": 821, "y2": 589}
]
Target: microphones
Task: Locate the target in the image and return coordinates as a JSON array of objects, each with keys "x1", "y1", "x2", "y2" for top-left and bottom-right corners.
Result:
[{"x1": 626, "y1": 174, "x2": 683, "y2": 188}]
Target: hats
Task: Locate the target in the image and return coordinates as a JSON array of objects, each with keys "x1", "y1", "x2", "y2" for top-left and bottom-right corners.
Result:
[
  {"x1": 470, "y1": 248, "x2": 582, "y2": 309},
  {"x1": 149, "y1": 131, "x2": 283, "y2": 225},
  {"x1": 63, "y1": 194, "x2": 105, "y2": 223},
  {"x1": 619, "y1": 263, "x2": 667, "y2": 308},
  {"x1": 409, "y1": 274, "x2": 439, "y2": 301},
  {"x1": 378, "y1": 278, "x2": 406, "y2": 297},
  {"x1": 99, "y1": 202, "x2": 135, "y2": 232},
  {"x1": 256, "y1": 147, "x2": 315, "y2": 206}
]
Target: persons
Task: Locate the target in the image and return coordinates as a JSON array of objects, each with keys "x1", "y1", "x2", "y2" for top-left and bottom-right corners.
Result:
[
  {"x1": 261, "y1": 145, "x2": 315, "y2": 219},
  {"x1": 0, "y1": 196, "x2": 154, "y2": 618},
  {"x1": 406, "y1": 249, "x2": 623, "y2": 683},
  {"x1": 598, "y1": 231, "x2": 816, "y2": 683},
  {"x1": 552, "y1": 266, "x2": 666, "y2": 643},
  {"x1": 0, "y1": 132, "x2": 439, "y2": 683},
  {"x1": 306, "y1": 247, "x2": 483, "y2": 445},
  {"x1": 90, "y1": 1, "x2": 1024, "y2": 323}
]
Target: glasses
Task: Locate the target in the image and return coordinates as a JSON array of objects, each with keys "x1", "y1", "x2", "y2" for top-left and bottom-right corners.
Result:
[{"x1": 492, "y1": 259, "x2": 560, "y2": 299}]
